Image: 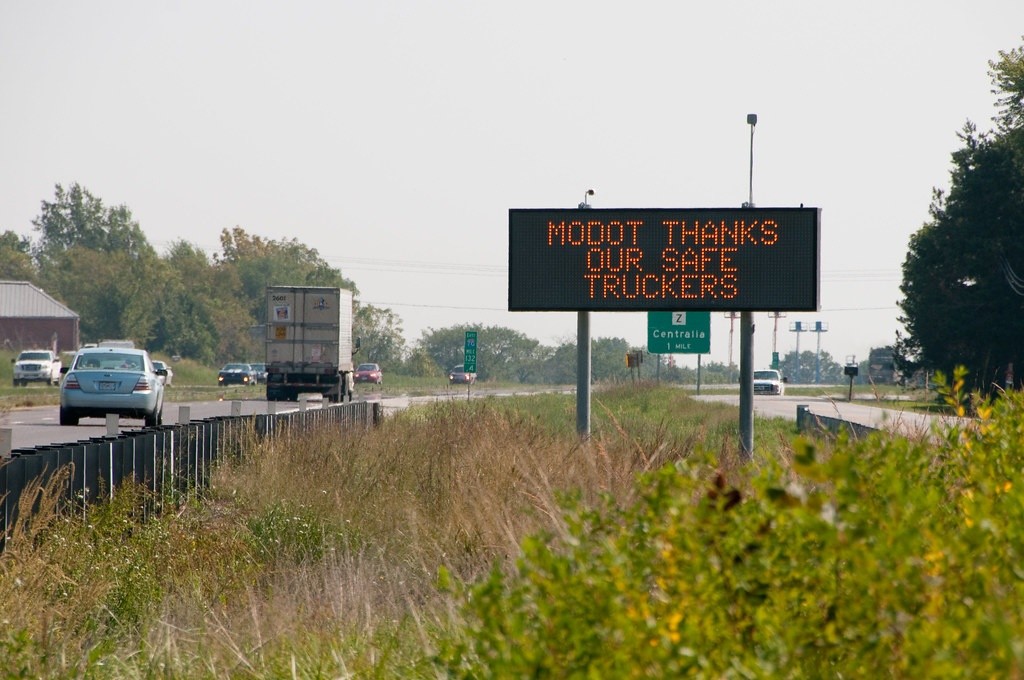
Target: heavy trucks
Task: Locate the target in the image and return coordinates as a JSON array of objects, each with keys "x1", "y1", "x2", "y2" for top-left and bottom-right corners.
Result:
[{"x1": 266, "y1": 286, "x2": 361, "y2": 402}]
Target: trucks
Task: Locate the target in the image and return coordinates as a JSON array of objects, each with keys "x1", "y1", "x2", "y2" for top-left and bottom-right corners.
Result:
[{"x1": 869, "y1": 348, "x2": 903, "y2": 386}]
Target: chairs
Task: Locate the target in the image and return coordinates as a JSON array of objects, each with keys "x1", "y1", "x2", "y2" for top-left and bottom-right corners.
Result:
[{"x1": 87, "y1": 358, "x2": 101, "y2": 367}]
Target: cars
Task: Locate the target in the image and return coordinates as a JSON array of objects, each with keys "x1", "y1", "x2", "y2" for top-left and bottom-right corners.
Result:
[
  {"x1": 250, "y1": 363, "x2": 268, "y2": 384},
  {"x1": 449, "y1": 364, "x2": 477, "y2": 384},
  {"x1": 11, "y1": 350, "x2": 62, "y2": 386},
  {"x1": 753, "y1": 370, "x2": 788, "y2": 396},
  {"x1": 218, "y1": 363, "x2": 257, "y2": 386},
  {"x1": 58, "y1": 348, "x2": 167, "y2": 427},
  {"x1": 152, "y1": 360, "x2": 174, "y2": 386},
  {"x1": 354, "y1": 363, "x2": 382, "y2": 385}
]
